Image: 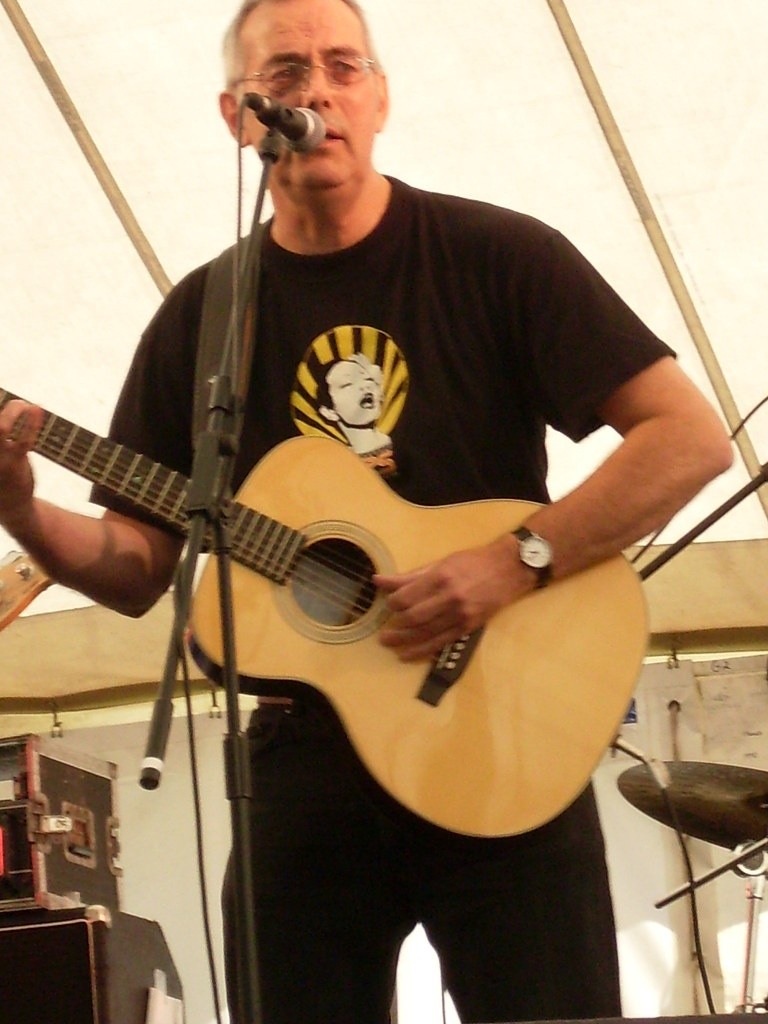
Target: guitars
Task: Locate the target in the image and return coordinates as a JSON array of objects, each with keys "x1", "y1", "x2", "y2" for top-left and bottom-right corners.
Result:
[
  {"x1": 0, "y1": 550, "x2": 57, "y2": 630},
  {"x1": 0, "y1": 387, "x2": 650, "y2": 838}
]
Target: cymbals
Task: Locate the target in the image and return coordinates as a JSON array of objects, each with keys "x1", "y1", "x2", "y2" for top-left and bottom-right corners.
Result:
[{"x1": 616, "y1": 760, "x2": 768, "y2": 851}]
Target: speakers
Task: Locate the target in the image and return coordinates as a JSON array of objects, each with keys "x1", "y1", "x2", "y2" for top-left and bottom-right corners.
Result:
[{"x1": 0, "y1": 904, "x2": 185, "y2": 1024}]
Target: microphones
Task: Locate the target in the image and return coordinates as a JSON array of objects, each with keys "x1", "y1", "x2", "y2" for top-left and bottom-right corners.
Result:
[{"x1": 245, "y1": 92, "x2": 326, "y2": 152}]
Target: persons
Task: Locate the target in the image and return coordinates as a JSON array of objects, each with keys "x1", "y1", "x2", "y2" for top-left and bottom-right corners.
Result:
[{"x1": 0, "y1": 0, "x2": 736, "y2": 1023}]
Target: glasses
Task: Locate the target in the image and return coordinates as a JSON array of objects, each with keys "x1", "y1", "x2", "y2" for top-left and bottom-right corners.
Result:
[{"x1": 233, "y1": 54, "x2": 376, "y2": 96}]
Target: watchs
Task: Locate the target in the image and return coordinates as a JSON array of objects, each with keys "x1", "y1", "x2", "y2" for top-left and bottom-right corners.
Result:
[{"x1": 510, "y1": 527, "x2": 553, "y2": 591}]
